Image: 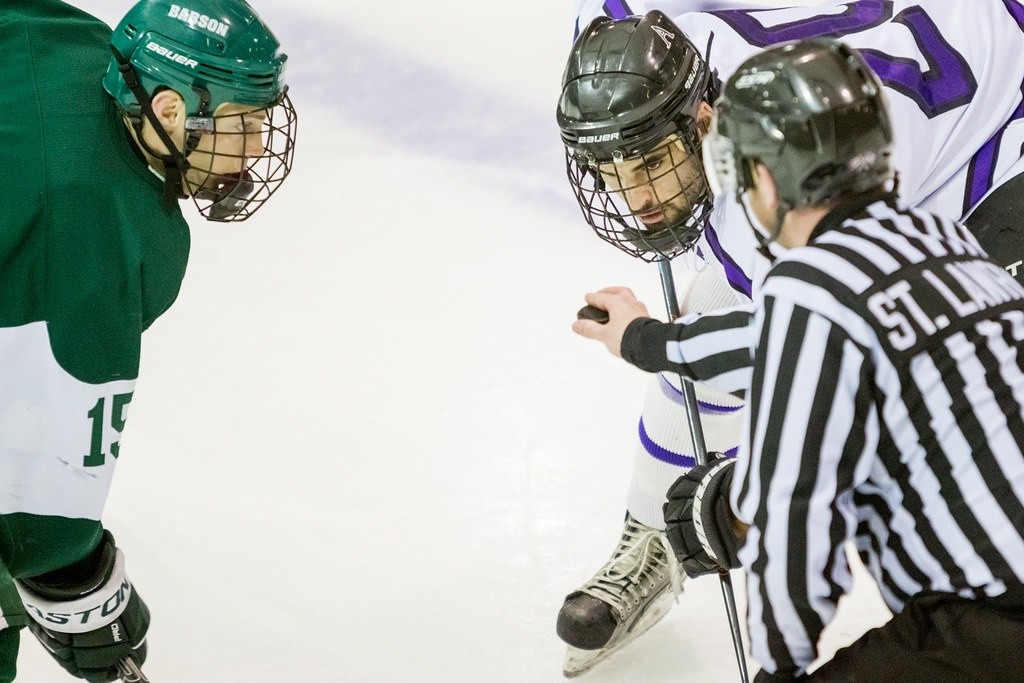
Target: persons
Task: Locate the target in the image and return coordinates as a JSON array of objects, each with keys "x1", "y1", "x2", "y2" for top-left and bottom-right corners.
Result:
[
  {"x1": 546, "y1": 0, "x2": 1024, "y2": 674},
  {"x1": 570, "y1": 37, "x2": 1024, "y2": 683},
  {"x1": 0, "y1": 2, "x2": 300, "y2": 683}
]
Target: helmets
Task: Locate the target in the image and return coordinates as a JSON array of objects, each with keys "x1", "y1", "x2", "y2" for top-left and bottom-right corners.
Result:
[
  {"x1": 102, "y1": 1, "x2": 298, "y2": 223},
  {"x1": 556, "y1": 10, "x2": 722, "y2": 264},
  {"x1": 702, "y1": 35, "x2": 895, "y2": 208}
]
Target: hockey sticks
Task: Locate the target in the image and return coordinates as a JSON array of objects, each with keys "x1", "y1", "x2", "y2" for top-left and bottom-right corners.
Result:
[
  {"x1": 112, "y1": 653, "x2": 150, "y2": 683},
  {"x1": 656, "y1": 252, "x2": 749, "y2": 682}
]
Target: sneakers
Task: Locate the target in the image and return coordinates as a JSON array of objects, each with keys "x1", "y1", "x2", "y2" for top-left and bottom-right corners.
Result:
[{"x1": 556, "y1": 509, "x2": 689, "y2": 680}]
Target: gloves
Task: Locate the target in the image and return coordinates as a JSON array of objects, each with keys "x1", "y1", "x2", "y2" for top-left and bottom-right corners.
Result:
[
  {"x1": 11, "y1": 529, "x2": 150, "y2": 683},
  {"x1": 663, "y1": 451, "x2": 745, "y2": 579}
]
